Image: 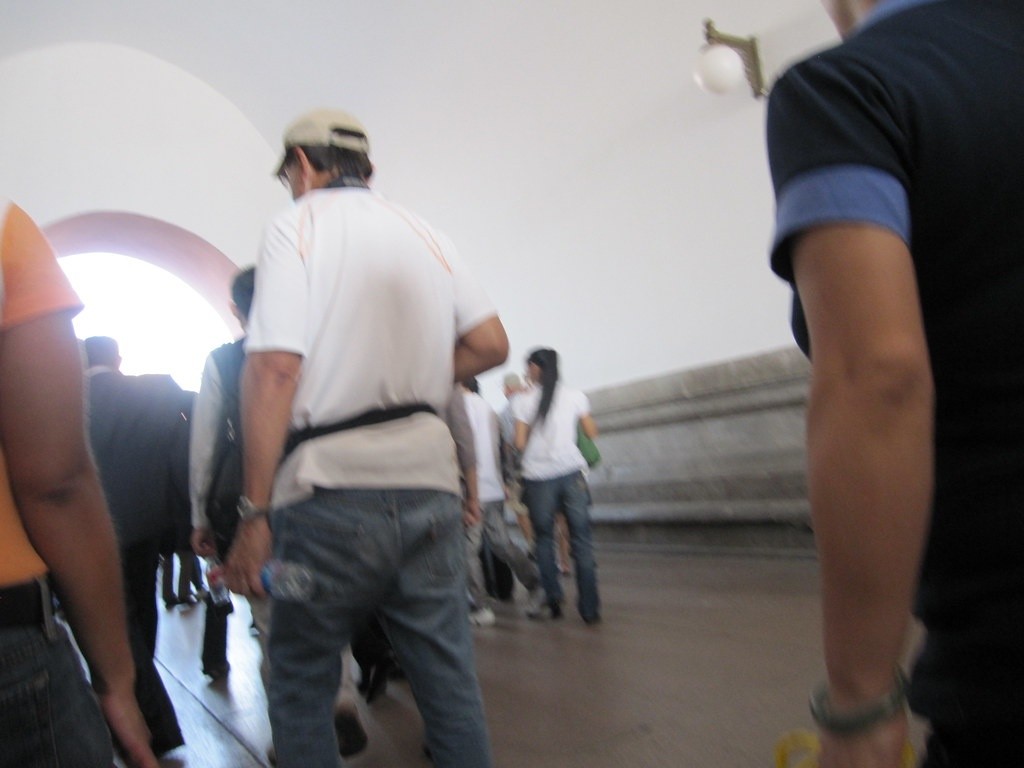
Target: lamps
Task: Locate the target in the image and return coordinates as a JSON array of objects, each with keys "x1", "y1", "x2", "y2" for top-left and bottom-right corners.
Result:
[{"x1": 698, "y1": 17, "x2": 768, "y2": 100}]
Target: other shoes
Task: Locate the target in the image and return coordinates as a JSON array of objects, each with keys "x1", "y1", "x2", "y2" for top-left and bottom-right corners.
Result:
[
  {"x1": 525, "y1": 578, "x2": 548, "y2": 618},
  {"x1": 165, "y1": 596, "x2": 178, "y2": 609},
  {"x1": 335, "y1": 708, "x2": 371, "y2": 760},
  {"x1": 178, "y1": 592, "x2": 200, "y2": 606},
  {"x1": 205, "y1": 660, "x2": 232, "y2": 679},
  {"x1": 527, "y1": 602, "x2": 559, "y2": 620},
  {"x1": 575, "y1": 598, "x2": 602, "y2": 627},
  {"x1": 470, "y1": 607, "x2": 497, "y2": 627}
]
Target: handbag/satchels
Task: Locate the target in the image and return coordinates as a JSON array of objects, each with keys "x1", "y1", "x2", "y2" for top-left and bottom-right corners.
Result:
[{"x1": 500, "y1": 440, "x2": 524, "y2": 486}]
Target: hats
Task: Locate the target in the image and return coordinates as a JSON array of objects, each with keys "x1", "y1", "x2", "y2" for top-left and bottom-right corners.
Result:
[{"x1": 273, "y1": 110, "x2": 370, "y2": 178}]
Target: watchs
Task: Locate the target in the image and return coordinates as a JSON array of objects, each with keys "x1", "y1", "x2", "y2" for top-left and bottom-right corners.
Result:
[{"x1": 236, "y1": 495, "x2": 268, "y2": 521}]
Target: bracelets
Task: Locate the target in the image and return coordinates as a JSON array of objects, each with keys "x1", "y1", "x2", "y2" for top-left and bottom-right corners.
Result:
[{"x1": 808, "y1": 659, "x2": 911, "y2": 732}]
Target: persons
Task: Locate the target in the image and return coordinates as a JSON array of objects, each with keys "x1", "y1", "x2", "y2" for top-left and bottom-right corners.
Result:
[
  {"x1": 766, "y1": 0, "x2": 1024, "y2": 768},
  {"x1": 54, "y1": 268, "x2": 406, "y2": 757},
  {"x1": 222, "y1": 109, "x2": 509, "y2": 768},
  {"x1": 445, "y1": 350, "x2": 602, "y2": 627},
  {"x1": 0, "y1": 190, "x2": 161, "y2": 768}
]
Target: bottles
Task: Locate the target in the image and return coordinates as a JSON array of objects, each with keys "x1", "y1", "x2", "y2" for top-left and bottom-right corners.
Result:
[
  {"x1": 258, "y1": 558, "x2": 315, "y2": 603},
  {"x1": 203, "y1": 543, "x2": 232, "y2": 607}
]
